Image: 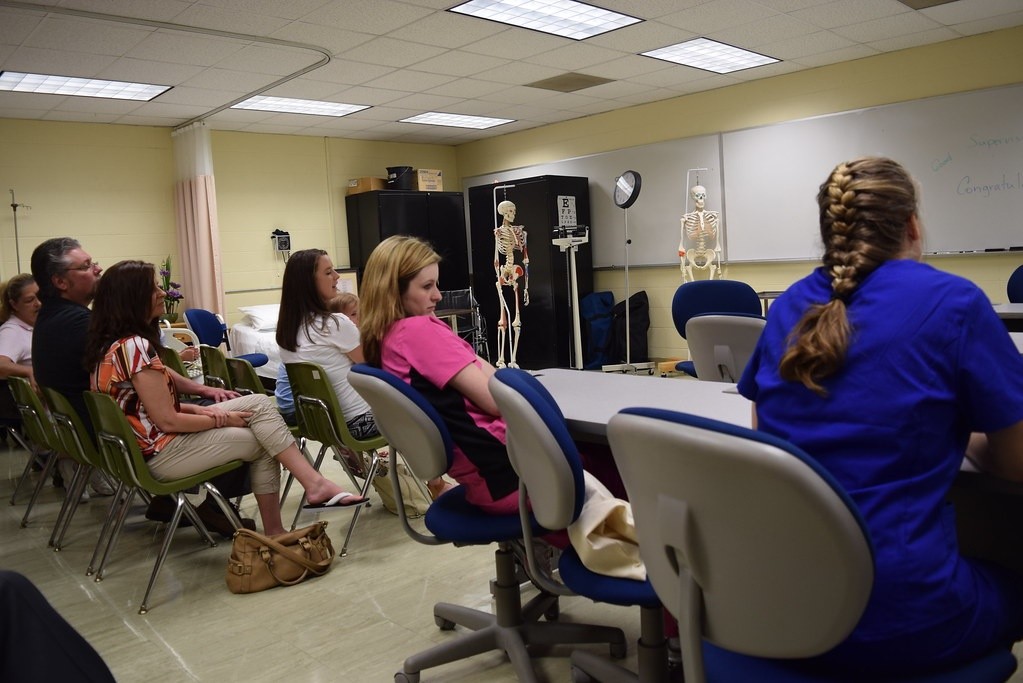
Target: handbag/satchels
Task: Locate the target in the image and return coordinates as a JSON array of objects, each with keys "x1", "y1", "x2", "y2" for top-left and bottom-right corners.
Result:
[
  {"x1": 226, "y1": 520, "x2": 335, "y2": 594},
  {"x1": 364, "y1": 457, "x2": 434, "y2": 518}
]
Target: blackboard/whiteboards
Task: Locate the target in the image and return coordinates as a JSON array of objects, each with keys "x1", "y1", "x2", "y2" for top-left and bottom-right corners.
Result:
[
  {"x1": 720, "y1": 82, "x2": 1023, "y2": 265},
  {"x1": 462, "y1": 132, "x2": 726, "y2": 271}
]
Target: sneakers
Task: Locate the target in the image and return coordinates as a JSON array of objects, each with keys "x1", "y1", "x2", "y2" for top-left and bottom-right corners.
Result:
[
  {"x1": 59, "y1": 458, "x2": 89, "y2": 503},
  {"x1": 88, "y1": 467, "x2": 118, "y2": 496}
]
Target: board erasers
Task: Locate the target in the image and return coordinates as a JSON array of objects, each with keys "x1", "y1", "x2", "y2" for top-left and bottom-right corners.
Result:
[{"x1": 1009, "y1": 246, "x2": 1023, "y2": 251}]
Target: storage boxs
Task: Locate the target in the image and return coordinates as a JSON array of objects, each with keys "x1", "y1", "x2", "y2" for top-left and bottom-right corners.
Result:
[
  {"x1": 412, "y1": 169, "x2": 444, "y2": 191},
  {"x1": 348, "y1": 177, "x2": 388, "y2": 194}
]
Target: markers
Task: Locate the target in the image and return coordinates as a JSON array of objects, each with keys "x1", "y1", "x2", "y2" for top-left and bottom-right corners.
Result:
[
  {"x1": 985, "y1": 248, "x2": 1005, "y2": 252},
  {"x1": 959, "y1": 251, "x2": 976, "y2": 253},
  {"x1": 934, "y1": 252, "x2": 949, "y2": 254}
]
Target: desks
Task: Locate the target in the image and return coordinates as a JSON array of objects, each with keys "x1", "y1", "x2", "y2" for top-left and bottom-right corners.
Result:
[
  {"x1": 527, "y1": 368, "x2": 1023, "y2": 496},
  {"x1": 991, "y1": 303, "x2": 1023, "y2": 333},
  {"x1": 160, "y1": 322, "x2": 193, "y2": 343},
  {"x1": 436, "y1": 308, "x2": 474, "y2": 335}
]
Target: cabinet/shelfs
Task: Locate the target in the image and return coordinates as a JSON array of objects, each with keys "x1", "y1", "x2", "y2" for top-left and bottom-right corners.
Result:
[
  {"x1": 344, "y1": 189, "x2": 469, "y2": 297},
  {"x1": 469, "y1": 174, "x2": 593, "y2": 369}
]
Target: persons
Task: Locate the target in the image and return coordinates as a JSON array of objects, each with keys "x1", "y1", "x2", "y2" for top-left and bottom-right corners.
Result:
[
  {"x1": 700, "y1": 156, "x2": 1023, "y2": 683},
  {"x1": 82, "y1": 260, "x2": 370, "y2": 538},
  {"x1": 0, "y1": 273, "x2": 117, "y2": 501},
  {"x1": 31, "y1": 237, "x2": 205, "y2": 452},
  {"x1": 276, "y1": 249, "x2": 456, "y2": 500},
  {"x1": 275, "y1": 361, "x2": 367, "y2": 479},
  {"x1": 357, "y1": 235, "x2": 683, "y2": 668},
  {"x1": 329, "y1": 293, "x2": 360, "y2": 323}
]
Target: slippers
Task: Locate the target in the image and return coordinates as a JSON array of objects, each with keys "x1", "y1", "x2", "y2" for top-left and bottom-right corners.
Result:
[{"x1": 303, "y1": 492, "x2": 370, "y2": 508}]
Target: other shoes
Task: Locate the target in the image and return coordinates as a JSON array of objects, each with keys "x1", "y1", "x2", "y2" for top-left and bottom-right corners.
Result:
[
  {"x1": 665, "y1": 643, "x2": 682, "y2": 669},
  {"x1": 332, "y1": 447, "x2": 351, "y2": 461},
  {"x1": 193, "y1": 501, "x2": 256, "y2": 539},
  {"x1": 427, "y1": 476, "x2": 454, "y2": 501},
  {"x1": 145, "y1": 498, "x2": 195, "y2": 529}
]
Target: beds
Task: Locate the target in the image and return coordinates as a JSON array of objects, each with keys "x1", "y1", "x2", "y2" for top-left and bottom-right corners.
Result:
[{"x1": 230, "y1": 322, "x2": 280, "y2": 395}]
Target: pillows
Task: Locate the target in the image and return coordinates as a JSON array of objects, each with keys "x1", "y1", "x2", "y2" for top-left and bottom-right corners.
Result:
[{"x1": 238, "y1": 304, "x2": 281, "y2": 332}]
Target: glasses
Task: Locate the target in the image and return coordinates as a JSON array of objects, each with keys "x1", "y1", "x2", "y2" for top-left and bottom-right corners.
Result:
[{"x1": 56, "y1": 261, "x2": 99, "y2": 271}]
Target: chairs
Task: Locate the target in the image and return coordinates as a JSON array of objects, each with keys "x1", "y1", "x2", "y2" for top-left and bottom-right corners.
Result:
[{"x1": 0, "y1": 265, "x2": 1023, "y2": 683}]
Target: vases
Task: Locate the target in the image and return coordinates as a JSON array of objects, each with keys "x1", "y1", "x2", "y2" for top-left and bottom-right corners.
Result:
[{"x1": 157, "y1": 312, "x2": 178, "y2": 323}]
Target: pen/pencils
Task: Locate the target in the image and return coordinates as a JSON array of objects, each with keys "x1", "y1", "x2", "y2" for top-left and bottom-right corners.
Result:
[{"x1": 722, "y1": 390, "x2": 739, "y2": 394}]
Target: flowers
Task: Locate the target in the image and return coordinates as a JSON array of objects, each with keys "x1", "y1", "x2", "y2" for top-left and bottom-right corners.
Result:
[{"x1": 158, "y1": 254, "x2": 185, "y2": 313}]
